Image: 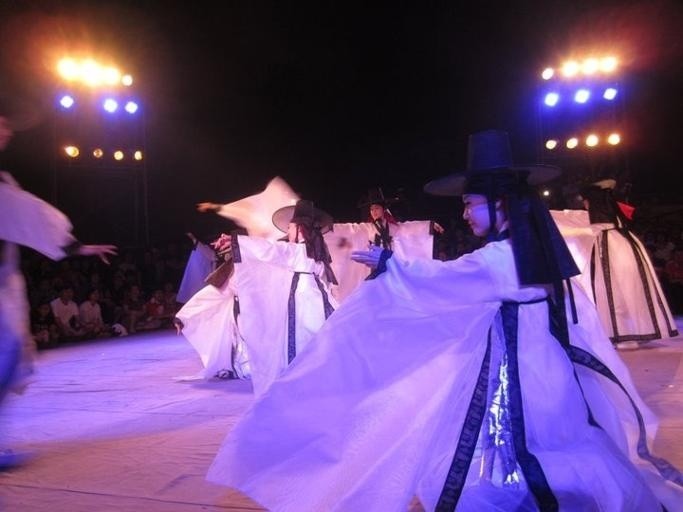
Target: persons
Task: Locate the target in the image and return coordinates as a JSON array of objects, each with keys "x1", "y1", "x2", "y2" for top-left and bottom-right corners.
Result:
[
  {"x1": 0, "y1": 94, "x2": 121, "y2": 479},
  {"x1": 208, "y1": 130, "x2": 681, "y2": 511},
  {"x1": 0, "y1": 244, "x2": 184, "y2": 353},
  {"x1": 535, "y1": 173, "x2": 681, "y2": 349},
  {"x1": 174, "y1": 176, "x2": 492, "y2": 394}
]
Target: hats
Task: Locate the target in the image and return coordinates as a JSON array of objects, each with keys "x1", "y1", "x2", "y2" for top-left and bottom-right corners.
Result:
[
  {"x1": 423, "y1": 130, "x2": 564, "y2": 197},
  {"x1": 271, "y1": 199, "x2": 334, "y2": 235},
  {"x1": 357, "y1": 186, "x2": 395, "y2": 208},
  {"x1": 0, "y1": 92, "x2": 38, "y2": 132},
  {"x1": 562, "y1": 165, "x2": 617, "y2": 191}
]
